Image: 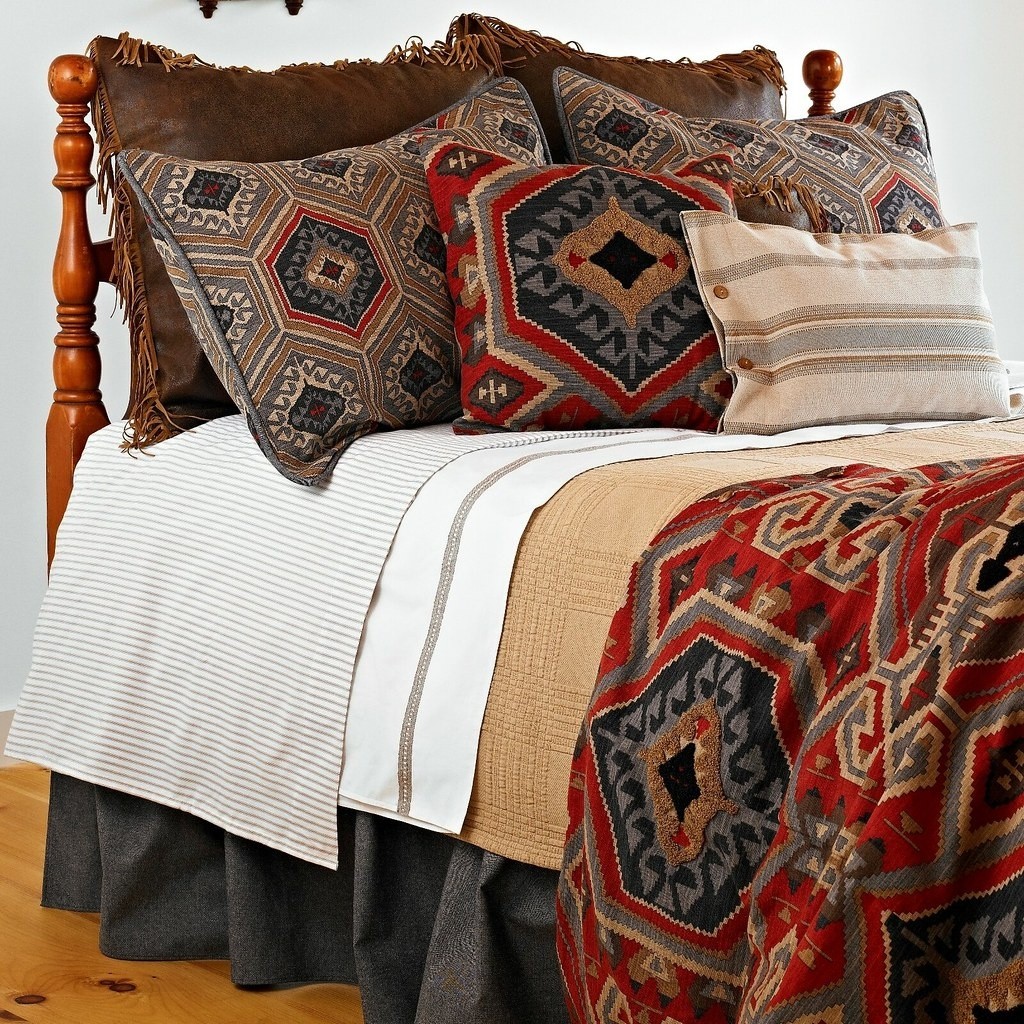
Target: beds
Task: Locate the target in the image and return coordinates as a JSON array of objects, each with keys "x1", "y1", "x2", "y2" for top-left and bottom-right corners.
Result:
[{"x1": 44, "y1": 52, "x2": 1024, "y2": 1023}]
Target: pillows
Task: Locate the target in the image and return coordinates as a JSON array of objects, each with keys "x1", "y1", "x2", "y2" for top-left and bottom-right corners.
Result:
[
  {"x1": 116, "y1": 82, "x2": 550, "y2": 486},
  {"x1": 456, "y1": 15, "x2": 789, "y2": 123},
  {"x1": 89, "y1": 37, "x2": 486, "y2": 445},
  {"x1": 678, "y1": 211, "x2": 1012, "y2": 436},
  {"x1": 552, "y1": 66, "x2": 944, "y2": 234},
  {"x1": 427, "y1": 143, "x2": 735, "y2": 431}
]
